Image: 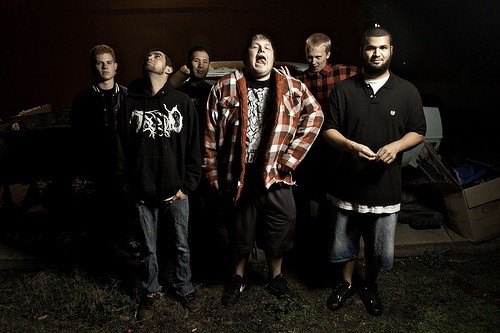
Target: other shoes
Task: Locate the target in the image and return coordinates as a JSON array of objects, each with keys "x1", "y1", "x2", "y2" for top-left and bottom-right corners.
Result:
[
  {"x1": 182, "y1": 293, "x2": 201, "y2": 312},
  {"x1": 139, "y1": 307, "x2": 154, "y2": 320}
]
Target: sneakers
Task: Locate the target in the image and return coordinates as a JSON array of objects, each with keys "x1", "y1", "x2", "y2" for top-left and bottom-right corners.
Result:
[
  {"x1": 326, "y1": 279, "x2": 353, "y2": 309},
  {"x1": 227, "y1": 274, "x2": 249, "y2": 297},
  {"x1": 269, "y1": 273, "x2": 290, "y2": 294},
  {"x1": 363, "y1": 284, "x2": 385, "y2": 316}
]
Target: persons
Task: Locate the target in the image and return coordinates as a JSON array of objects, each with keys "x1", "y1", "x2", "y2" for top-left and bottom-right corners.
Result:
[
  {"x1": 107, "y1": 42, "x2": 203, "y2": 321},
  {"x1": 174, "y1": 47, "x2": 219, "y2": 281},
  {"x1": 278, "y1": 32, "x2": 358, "y2": 273},
  {"x1": 321, "y1": 28, "x2": 427, "y2": 315},
  {"x1": 200, "y1": 34, "x2": 324, "y2": 304},
  {"x1": 70, "y1": 46, "x2": 128, "y2": 277}
]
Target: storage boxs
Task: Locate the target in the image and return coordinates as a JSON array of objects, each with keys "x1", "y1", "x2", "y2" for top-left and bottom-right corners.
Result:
[{"x1": 443, "y1": 177, "x2": 500, "y2": 243}]
